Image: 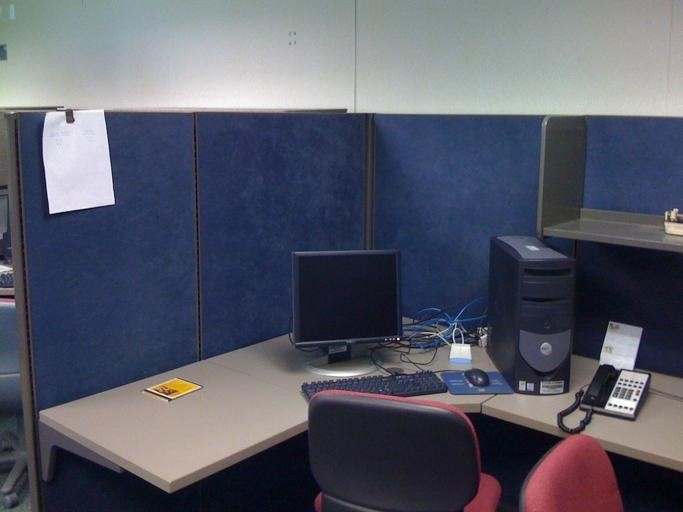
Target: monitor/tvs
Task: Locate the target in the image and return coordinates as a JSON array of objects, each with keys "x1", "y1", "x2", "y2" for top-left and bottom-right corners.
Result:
[{"x1": 291, "y1": 248, "x2": 403, "y2": 378}]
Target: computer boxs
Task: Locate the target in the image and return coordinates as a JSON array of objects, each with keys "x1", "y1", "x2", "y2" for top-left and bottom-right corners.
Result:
[{"x1": 487, "y1": 235, "x2": 577, "y2": 394}]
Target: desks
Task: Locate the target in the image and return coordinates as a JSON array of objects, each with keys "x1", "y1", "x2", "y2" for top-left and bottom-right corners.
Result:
[
  {"x1": 37, "y1": 315, "x2": 505, "y2": 492},
  {"x1": 481, "y1": 348, "x2": 683, "y2": 473}
]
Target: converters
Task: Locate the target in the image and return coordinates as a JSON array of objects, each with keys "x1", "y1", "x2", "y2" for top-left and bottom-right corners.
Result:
[{"x1": 448, "y1": 343, "x2": 472, "y2": 363}]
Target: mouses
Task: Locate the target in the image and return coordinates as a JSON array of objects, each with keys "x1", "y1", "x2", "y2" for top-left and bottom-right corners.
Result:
[{"x1": 464, "y1": 367, "x2": 489, "y2": 386}]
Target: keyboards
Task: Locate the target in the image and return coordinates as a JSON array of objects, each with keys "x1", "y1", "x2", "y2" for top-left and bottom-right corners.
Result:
[{"x1": 301, "y1": 370, "x2": 448, "y2": 404}]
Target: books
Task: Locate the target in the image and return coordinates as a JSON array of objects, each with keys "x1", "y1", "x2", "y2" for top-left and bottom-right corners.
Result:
[{"x1": 141, "y1": 377, "x2": 203, "y2": 403}]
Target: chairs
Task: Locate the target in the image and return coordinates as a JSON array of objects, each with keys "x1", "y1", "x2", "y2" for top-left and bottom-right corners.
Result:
[
  {"x1": 305, "y1": 392, "x2": 502, "y2": 512},
  {"x1": 519, "y1": 434, "x2": 624, "y2": 512}
]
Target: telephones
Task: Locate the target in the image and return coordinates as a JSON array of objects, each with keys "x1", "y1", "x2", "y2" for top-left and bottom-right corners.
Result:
[{"x1": 579, "y1": 364, "x2": 650, "y2": 420}]
